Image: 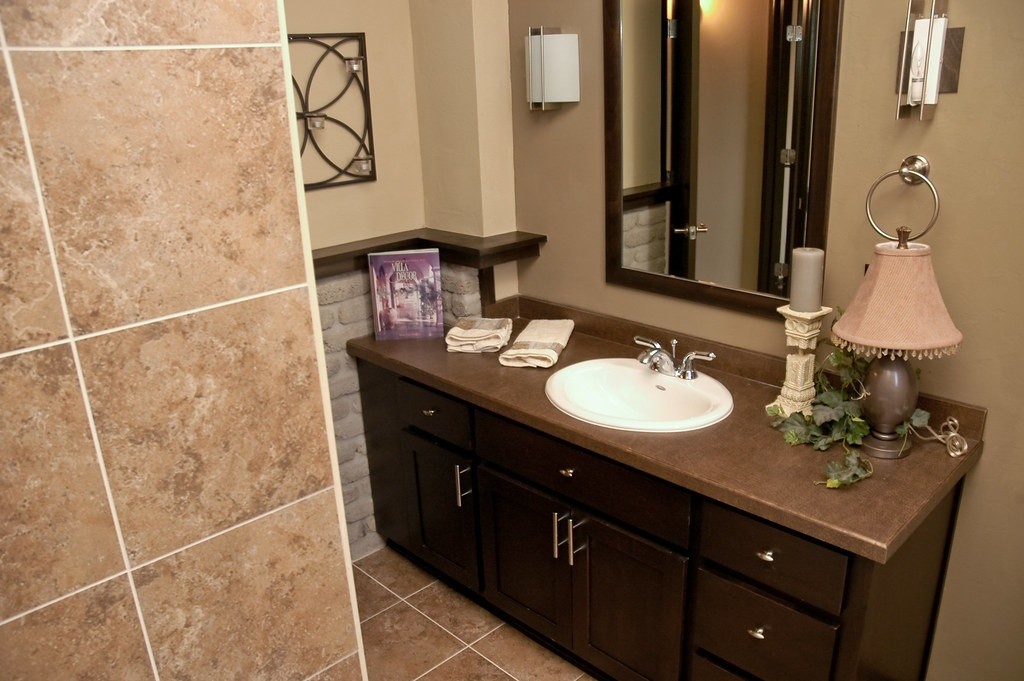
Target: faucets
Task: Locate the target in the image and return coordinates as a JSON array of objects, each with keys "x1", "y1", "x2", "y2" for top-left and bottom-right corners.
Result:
[{"x1": 638, "y1": 348, "x2": 681, "y2": 376}]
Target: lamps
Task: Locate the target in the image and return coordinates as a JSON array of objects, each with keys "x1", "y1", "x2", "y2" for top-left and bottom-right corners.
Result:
[
  {"x1": 524, "y1": 26, "x2": 580, "y2": 111},
  {"x1": 831, "y1": 227, "x2": 961, "y2": 457},
  {"x1": 894, "y1": 0, "x2": 950, "y2": 122}
]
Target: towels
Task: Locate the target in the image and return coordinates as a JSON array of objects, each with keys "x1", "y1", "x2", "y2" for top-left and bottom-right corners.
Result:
[
  {"x1": 498, "y1": 319, "x2": 575, "y2": 368},
  {"x1": 445, "y1": 316, "x2": 513, "y2": 353}
]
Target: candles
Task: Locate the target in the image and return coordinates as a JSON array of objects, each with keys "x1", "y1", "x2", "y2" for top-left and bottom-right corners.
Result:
[
  {"x1": 790, "y1": 248, "x2": 824, "y2": 314},
  {"x1": 344, "y1": 56, "x2": 362, "y2": 74},
  {"x1": 305, "y1": 112, "x2": 325, "y2": 130},
  {"x1": 353, "y1": 155, "x2": 372, "y2": 173}
]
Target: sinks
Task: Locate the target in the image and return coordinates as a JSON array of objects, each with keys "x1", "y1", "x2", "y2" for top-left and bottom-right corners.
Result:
[{"x1": 545, "y1": 358, "x2": 734, "y2": 433}]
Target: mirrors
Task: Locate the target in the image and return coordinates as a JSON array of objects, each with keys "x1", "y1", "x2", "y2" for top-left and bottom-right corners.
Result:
[{"x1": 602, "y1": 0, "x2": 846, "y2": 322}]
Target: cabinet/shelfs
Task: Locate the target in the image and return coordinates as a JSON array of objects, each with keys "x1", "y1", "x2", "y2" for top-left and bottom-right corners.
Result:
[{"x1": 347, "y1": 296, "x2": 986, "y2": 681}]
